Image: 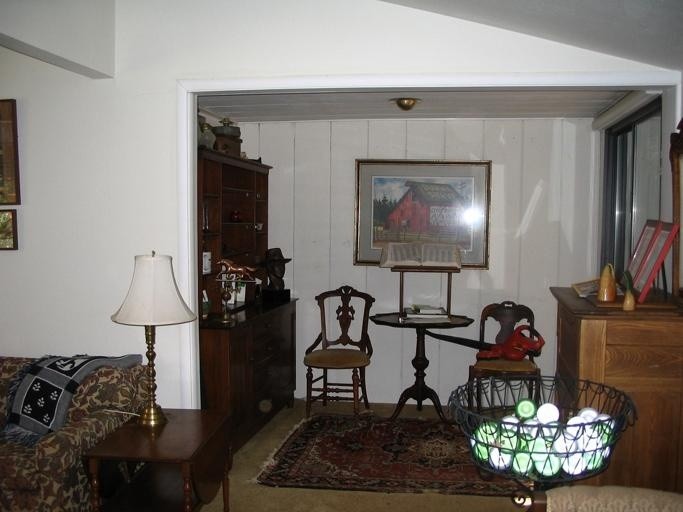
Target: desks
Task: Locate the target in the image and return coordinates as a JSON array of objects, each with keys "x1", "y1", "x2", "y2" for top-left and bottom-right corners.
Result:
[
  {"x1": 367, "y1": 310, "x2": 475, "y2": 428},
  {"x1": 79, "y1": 407, "x2": 236, "y2": 512}
]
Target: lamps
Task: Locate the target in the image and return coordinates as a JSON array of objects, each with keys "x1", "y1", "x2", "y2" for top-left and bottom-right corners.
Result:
[{"x1": 108, "y1": 249, "x2": 200, "y2": 427}]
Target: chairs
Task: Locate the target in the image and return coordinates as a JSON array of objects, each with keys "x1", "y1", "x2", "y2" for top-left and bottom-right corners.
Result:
[
  {"x1": 301, "y1": 284, "x2": 374, "y2": 420},
  {"x1": 466, "y1": 301, "x2": 540, "y2": 425},
  {"x1": 510, "y1": 481, "x2": 681, "y2": 512}
]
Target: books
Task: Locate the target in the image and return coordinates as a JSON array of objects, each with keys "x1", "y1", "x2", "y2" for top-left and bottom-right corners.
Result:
[{"x1": 571, "y1": 279, "x2": 624, "y2": 298}]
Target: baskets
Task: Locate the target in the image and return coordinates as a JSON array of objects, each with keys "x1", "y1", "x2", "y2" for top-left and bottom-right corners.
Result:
[{"x1": 449, "y1": 375, "x2": 635, "y2": 483}]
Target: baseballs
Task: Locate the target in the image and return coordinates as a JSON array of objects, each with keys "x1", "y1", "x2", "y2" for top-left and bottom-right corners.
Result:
[{"x1": 471, "y1": 399, "x2": 614, "y2": 477}]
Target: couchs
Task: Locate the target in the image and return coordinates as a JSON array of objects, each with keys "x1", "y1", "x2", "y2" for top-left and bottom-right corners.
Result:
[{"x1": 0, "y1": 355, "x2": 148, "y2": 512}]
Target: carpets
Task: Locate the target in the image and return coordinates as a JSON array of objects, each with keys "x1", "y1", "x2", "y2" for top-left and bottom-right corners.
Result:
[{"x1": 244, "y1": 410, "x2": 552, "y2": 500}]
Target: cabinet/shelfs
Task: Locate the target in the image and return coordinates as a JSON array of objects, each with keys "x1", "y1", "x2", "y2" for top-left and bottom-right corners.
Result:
[
  {"x1": 196, "y1": 143, "x2": 272, "y2": 321},
  {"x1": 199, "y1": 297, "x2": 300, "y2": 469},
  {"x1": 548, "y1": 286, "x2": 682, "y2": 492}
]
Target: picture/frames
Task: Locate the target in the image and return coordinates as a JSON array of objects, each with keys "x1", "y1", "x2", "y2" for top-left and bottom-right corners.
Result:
[
  {"x1": 631, "y1": 221, "x2": 679, "y2": 304},
  {"x1": 0, "y1": 208, "x2": 19, "y2": 252},
  {"x1": 0, "y1": 96, "x2": 21, "y2": 206},
  {"x1": 618, "y1": 218, "x2": 662, "y2": 297},
  {"x1": 352, "y1": 157, "x2": 492, "y2": 268}
]
